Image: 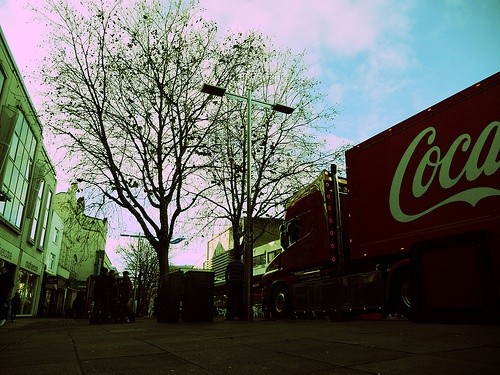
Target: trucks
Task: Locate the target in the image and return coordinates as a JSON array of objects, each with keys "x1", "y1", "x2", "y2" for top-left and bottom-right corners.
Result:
[
  {"x1": 153, "y1": 268, "x2": 215, "y2": 323},
  {"x1": 262, "y1": 70, "x2": 500, "y2": 327}
]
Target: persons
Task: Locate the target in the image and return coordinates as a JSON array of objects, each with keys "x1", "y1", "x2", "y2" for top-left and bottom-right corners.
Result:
[
  {"x1": 10, "y1": 292, "x2": 21, "y2": 322},
  {"x1": 223, "y1": 253, "x2": 244, "y2": 321},
  {"x1": 290, "y1": 224, "x2": 300, "y2": 242},
  {"x1": 89, "y1": 267, "x2": 135, "y2": 326},
  {"x1": 0, "y1": 260, "x2": 14, "y2": 326},
  {"x1": 73, "y1": 291, "x2": 84, "y2": 321}
]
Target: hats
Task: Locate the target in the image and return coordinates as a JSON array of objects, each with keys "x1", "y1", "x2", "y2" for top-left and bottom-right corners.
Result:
[{"x1": 100, "y1": 266, "x2": 108, "y2": 272}]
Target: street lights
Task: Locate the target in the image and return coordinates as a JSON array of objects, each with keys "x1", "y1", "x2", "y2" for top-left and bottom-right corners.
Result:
[
  {"x1": 199, "y1": 82, "x2": 297, "y2": 322},
  {"x1": 120, "y1": 232, "x2": 158, "y2": 319}
]
